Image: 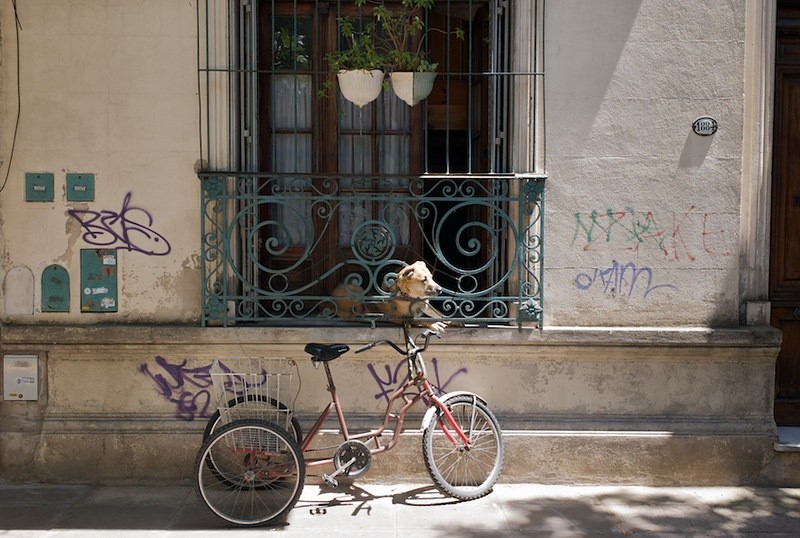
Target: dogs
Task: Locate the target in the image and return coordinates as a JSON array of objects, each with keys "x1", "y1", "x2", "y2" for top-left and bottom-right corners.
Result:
[{"x1": 330, "y1": 261, "x2": 465, "y2": 331}]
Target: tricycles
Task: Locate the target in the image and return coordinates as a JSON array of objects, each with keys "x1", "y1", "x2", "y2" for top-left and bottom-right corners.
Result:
[{"x1": 191, "y1": 315, "x2": 505, "y2": 528}]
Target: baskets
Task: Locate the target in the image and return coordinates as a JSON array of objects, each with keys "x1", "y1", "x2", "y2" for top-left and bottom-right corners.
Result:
[{"x1": 209, "y1": 358, "x2": 292, "y2": 451}]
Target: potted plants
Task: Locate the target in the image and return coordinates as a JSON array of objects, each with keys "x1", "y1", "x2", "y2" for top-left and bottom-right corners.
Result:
[
  {"x1": 356, "y1": 0, "x2": 473, "y2": 108},
  {"x1": 326, "y1": 13, "x2": 387, "y2": 107}
]
